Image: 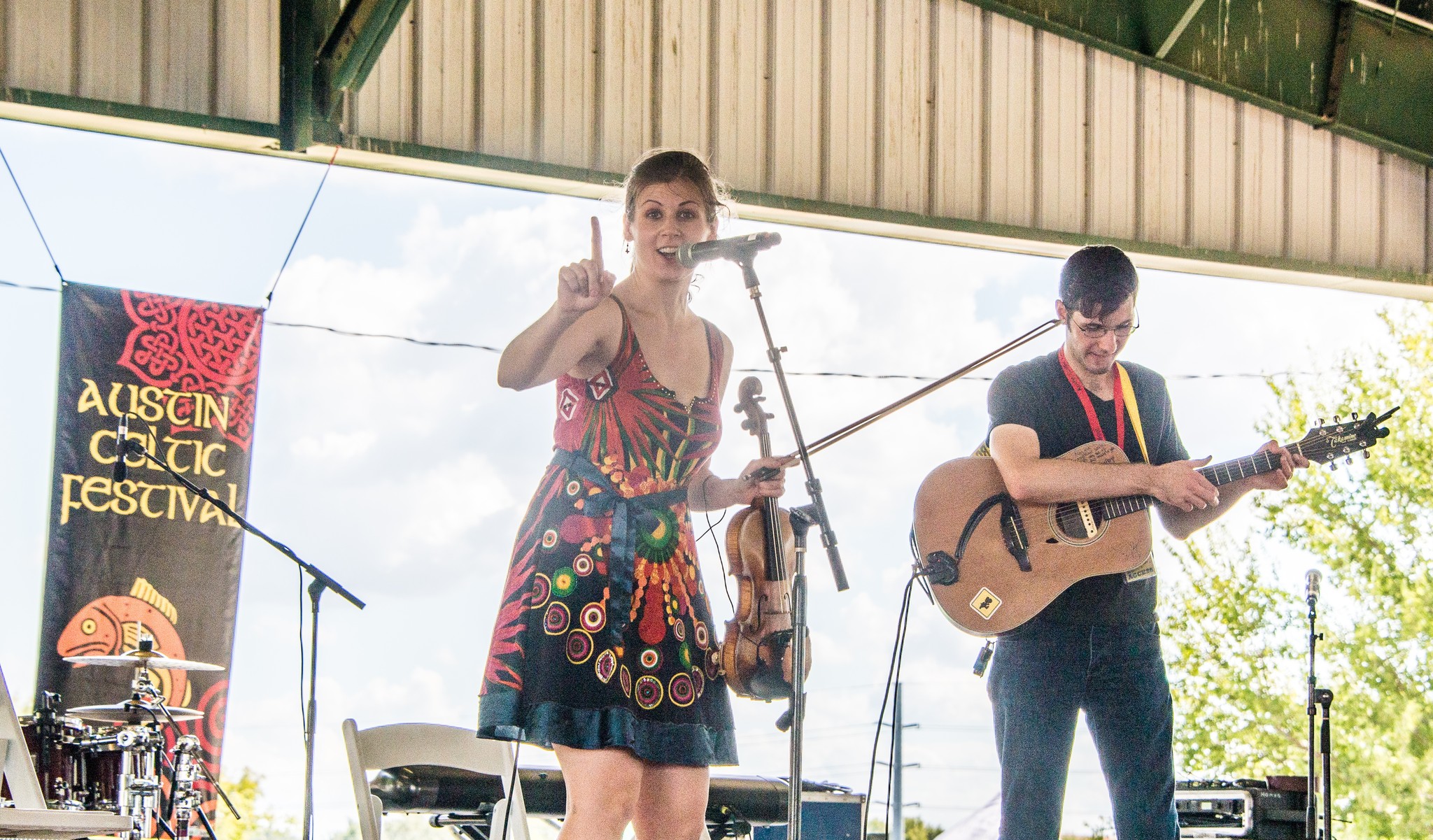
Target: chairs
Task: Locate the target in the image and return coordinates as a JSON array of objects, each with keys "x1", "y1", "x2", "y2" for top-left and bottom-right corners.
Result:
[
  {"x1": 340, "y1": 712, "x2": 536, "y2": 840},
  {"x1": 0, "y1": 665, "x2": 134, "y2": 840}
]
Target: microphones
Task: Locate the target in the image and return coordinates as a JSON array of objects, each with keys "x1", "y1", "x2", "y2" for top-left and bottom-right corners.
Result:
[
  {"x1": 674, "y1": 231, "x2": 781, "y2": 270},
  {"x1": 112, "y1": 414, "x2": 128, "y2": 483},
  {"x1": 1304, "y1": 570, "x2": 1323, "y2": 604}
]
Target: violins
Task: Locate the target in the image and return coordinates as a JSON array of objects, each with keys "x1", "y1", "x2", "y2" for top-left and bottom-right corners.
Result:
[{"x1": 722, "y1": 376, "x2": 811, "y2": 703}]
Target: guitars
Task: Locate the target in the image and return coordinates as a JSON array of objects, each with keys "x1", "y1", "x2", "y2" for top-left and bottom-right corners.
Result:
[{"x1": 912, "y1": 412, "x2": 1377, "y2": 637}]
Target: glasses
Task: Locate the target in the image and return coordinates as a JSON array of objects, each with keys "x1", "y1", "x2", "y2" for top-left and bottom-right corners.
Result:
[{"x1": 1062, "y1": 301, "x2": 1139, "y2": 339}]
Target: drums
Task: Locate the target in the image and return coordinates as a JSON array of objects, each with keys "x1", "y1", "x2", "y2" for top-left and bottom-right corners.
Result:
[
  {"x1": 0, "y1": 716, "x2": 81, "y2": 810},
  {"x1": 73, "y1": 726, "x2": 161, "y2": 814}
]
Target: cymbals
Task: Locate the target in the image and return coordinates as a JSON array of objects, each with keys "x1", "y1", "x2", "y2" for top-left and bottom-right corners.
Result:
[
  {"x1": 63, "y1": 697, "x2": 206, "y2": 724},
  {"x1": 62, "y1": 651, "x2": 225, "y2": 670}
]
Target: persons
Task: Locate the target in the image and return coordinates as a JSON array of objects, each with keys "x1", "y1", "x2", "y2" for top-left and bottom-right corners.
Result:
[
  {"x1": 985, "y1": 244, "x2": 1309, "y2": 840},
  {"x1": 467, "y1": 148, "x2": 799, "y2": 840}
]
